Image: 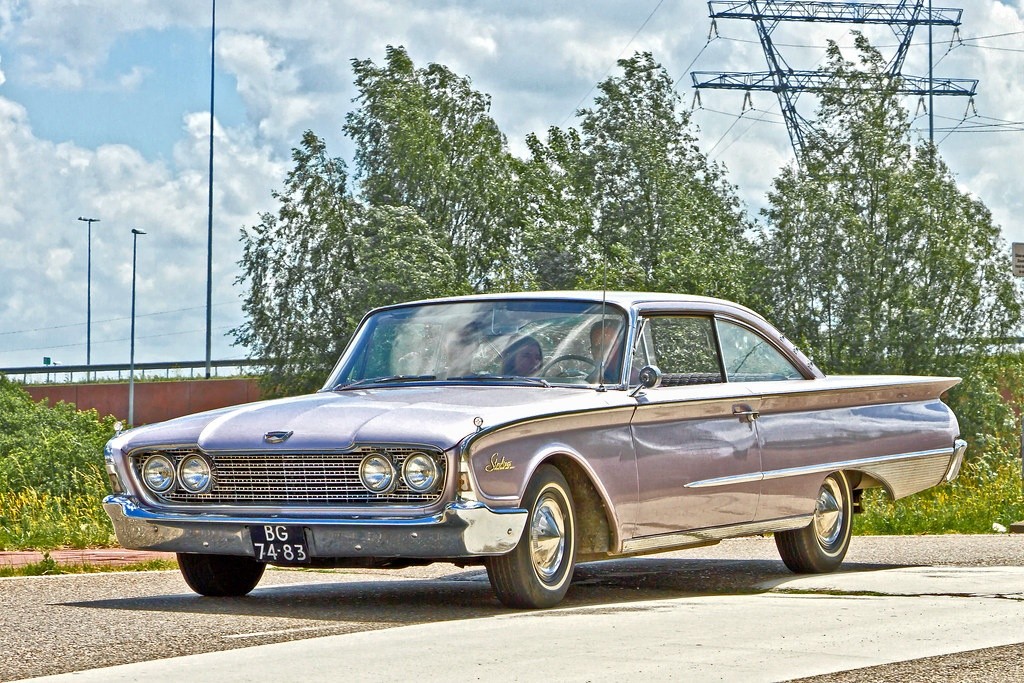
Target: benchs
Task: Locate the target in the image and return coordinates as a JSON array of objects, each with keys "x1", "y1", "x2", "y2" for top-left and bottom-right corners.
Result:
[{"x1": 661, "y1": 372, "x2": 788, "y2": 388}]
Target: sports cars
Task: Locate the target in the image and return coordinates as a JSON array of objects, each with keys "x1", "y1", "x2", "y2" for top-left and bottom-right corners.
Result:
[{"x1": 98, "y1": 286, "x2": 969, "y2": 612}]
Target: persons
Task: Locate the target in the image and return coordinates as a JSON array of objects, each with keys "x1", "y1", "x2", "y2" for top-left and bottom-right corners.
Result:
[
  {"x1": 585, "y1": 319, "x2": 641, "y2": 389},
  {"x1": 435, "y1": 316, "x2": 495, "y2": 377},
  {"x1": 501, "y1": 334, "x2": 544, "y2": 379}
]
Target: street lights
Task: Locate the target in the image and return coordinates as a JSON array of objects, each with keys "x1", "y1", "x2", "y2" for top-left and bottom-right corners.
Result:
[
  {"x1": 127, "y1": 228, "x2": 148, "y2": 430},
  {"x1": 76, "y1": 216, "x2": 102, "y2": 384}
]
